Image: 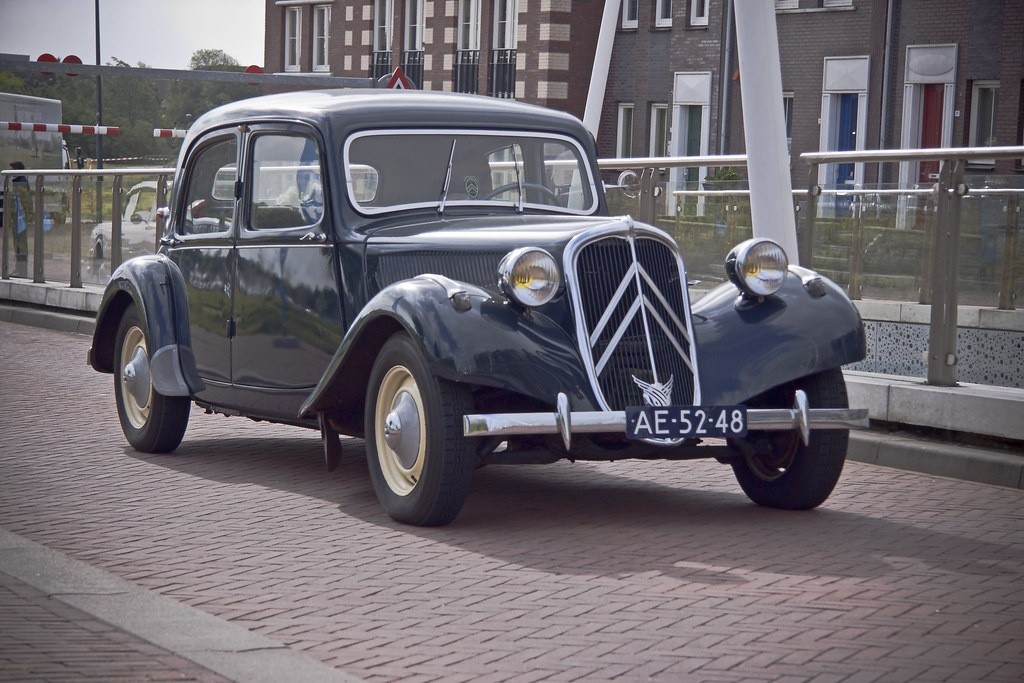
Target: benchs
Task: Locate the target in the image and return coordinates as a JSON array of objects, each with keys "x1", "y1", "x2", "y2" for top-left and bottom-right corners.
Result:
[{"x1": 208, "y1": 205, "x2": 295, "y2": 228}]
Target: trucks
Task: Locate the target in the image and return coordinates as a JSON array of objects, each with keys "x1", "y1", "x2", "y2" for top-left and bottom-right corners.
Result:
[{"x1": 0, "y1": 93, "x2": 83, "y2": 226}]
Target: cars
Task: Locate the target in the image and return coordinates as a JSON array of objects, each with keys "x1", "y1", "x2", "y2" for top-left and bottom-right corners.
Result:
[
  {"x1": 88, "y1": 180, "x2": 175, "y2": 285},
  {"x1": 85, "y1": 86, "x2": 870, "y2": 527}
]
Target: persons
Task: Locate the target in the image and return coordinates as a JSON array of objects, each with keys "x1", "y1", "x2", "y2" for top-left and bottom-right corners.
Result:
[{"x1": 7, "y1": 161, "x2": 34, "y2": 277}]
